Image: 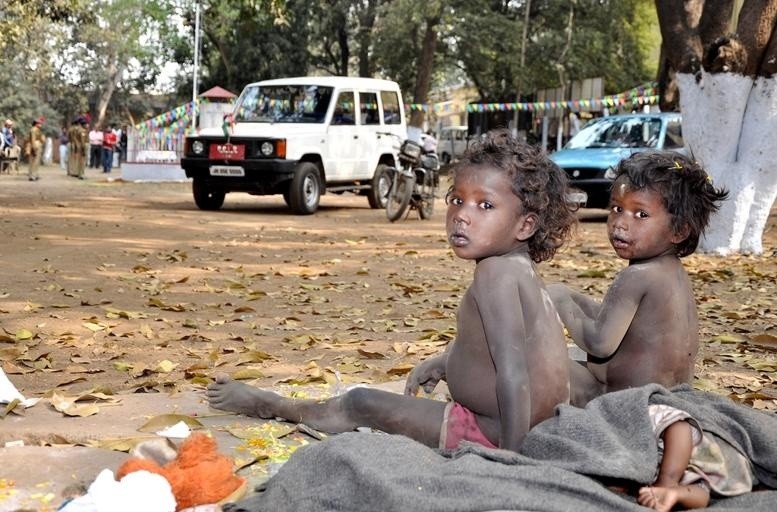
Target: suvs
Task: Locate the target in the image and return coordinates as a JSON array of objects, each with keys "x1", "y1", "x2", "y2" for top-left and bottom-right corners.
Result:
[{"x1": 180, "y1": 75, "x2": 409, "y2": 215}]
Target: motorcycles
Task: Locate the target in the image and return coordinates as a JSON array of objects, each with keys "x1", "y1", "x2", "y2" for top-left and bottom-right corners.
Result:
[{"x1": 384, "y1": 138, "x2": 442, "y2": 223}]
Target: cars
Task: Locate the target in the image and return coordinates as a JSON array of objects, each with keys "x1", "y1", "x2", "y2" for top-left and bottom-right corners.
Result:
[
  {"x1": 438, "y1": 125, "x2": 470, "y2": 164},
  {"x1": 546, "y1": 111, "x2": 683, "y2": 208}
]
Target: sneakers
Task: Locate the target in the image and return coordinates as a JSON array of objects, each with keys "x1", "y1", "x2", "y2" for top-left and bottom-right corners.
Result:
[{"x1": 117, "y1": 433, "x2": 246, "y2": 508}]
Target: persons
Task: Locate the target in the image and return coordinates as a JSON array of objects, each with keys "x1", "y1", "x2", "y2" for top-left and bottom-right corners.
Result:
[
  {"x1": 204, "y1": 130, "x2": 576, "y2": 455},
  {"x1": 418, "y1": 128, "x2": 438, "y2": 155},
  {"x1": 597, "y1": 402, "x2": 759, "y2": 512},
  {"x1": 544, "y1": 145, "x2": 733, "y2": 408},
  {"x1": 0, "y1": 114, "x2": 128, "y2": 180}
]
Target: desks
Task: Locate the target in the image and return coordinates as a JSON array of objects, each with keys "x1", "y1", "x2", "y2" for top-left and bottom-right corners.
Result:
[{"x1": 120, "y1": 160, "x2": 192, "y2": 182}]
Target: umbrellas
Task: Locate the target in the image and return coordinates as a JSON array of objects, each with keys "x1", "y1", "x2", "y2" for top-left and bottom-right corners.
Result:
[{"x1": 196, "y1": 86, "x2": 236, "y2": 105}]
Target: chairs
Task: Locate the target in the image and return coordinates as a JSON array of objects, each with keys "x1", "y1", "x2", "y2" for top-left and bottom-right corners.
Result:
[
  {"x1": 1, "y1": 146, "x2": 21, "y2": 175},
  {"x1": 303, "y1": 94, "x2": 377, "y2": 124}
]
